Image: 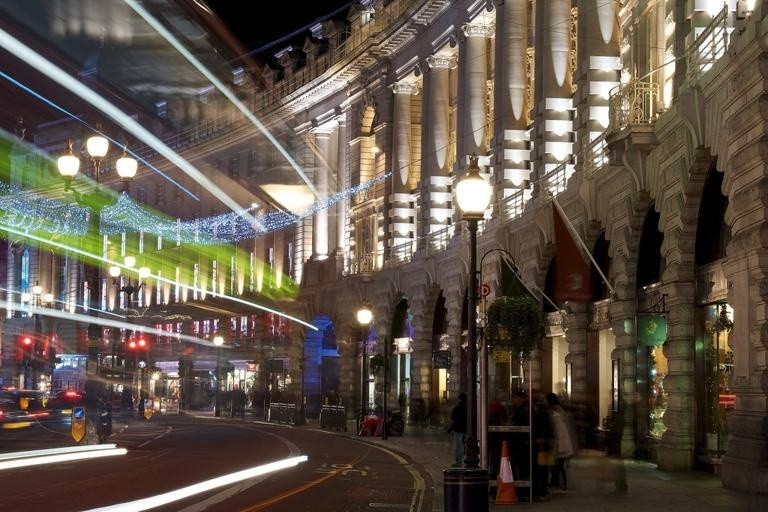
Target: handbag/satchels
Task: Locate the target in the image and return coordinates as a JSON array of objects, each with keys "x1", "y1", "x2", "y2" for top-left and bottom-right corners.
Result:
[{"x1": 538, "y1": 451, "x2": 555, "y2": 466}]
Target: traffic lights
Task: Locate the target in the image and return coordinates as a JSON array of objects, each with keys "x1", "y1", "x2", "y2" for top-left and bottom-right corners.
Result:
[
  {"x1": 128, "y1": 338, "x2": 145, "y2": 350},
  {"x1": 23, "y1": 337, "x2": 32, "y2": 344}
]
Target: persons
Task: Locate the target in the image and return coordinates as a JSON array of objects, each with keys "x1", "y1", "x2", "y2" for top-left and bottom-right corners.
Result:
[
  {"x1": 398, "y1": 391, "x2": 407, "y2": 411},
  {"x1": 475, "y1": 388, "x2": 574, "y2": 503},
  {"x1": 449, "y1": 391, "x2": 467, "y2": 468}
]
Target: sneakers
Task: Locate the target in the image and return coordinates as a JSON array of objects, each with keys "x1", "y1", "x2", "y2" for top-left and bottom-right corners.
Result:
[
  {"x1": 450, "y1": 463, "x2": 461, "y2": 468},
  {"x1": 535, "y1": 481, "x2": 567, "y2": 502}
]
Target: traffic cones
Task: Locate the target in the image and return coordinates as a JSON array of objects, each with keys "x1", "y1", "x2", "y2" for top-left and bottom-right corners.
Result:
[{"x1": 494, "y1": 441, "x2": 518, "y2": 505}]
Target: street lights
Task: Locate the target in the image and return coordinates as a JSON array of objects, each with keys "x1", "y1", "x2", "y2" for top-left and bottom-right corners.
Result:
[
  {"x1": 58, "y1": 124, "x2": 139, "y2": 435},
  {"x1": 109, "y1": 256, "x2": 149, "y2": 412},
  {"x1": 21, "y1": 281, "x2": 54, "y2": 390},
  {"x1": 456, "y1": 154, "x2": 492, "y2": 468},
  {"x1": 213, "y1": 335, "x2": 224, "y2": 415},
  {"x1": 357, "y1": 298, "x2": 372, "y2": 422}
]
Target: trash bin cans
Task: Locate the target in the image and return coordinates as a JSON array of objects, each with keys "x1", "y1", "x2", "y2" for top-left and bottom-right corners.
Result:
[
  {"x1": 269, "y1": 402, "x2": 296, "y2": 425},
  {"x1": 214, "y1": 405, "x2": 221, "y2": 416},
  {"x1": 321, "y1": 405, "x2": 345, "y2": 432},
  {"x1": 444, "y1": 467, "x2": 489, "y2": 512}
]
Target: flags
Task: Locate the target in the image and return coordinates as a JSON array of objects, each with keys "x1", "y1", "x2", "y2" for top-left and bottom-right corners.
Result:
[
  {"x1": 498, "y1": 254, "x2": 543, "y2": 307},
  {"x1": 548, "y1": 199, "x2": 596, "y2": 302}
]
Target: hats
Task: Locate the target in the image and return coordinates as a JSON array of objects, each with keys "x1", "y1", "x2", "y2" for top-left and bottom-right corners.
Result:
[
  {"x1": 488, "y1": 400, "x2": 504, "y2": 414},
  {"x1": 534, "y1": 397, "x2": 545, "y2": 407},
  {"x1": 459, "y1": 392, "x2": 467, "y2": 400},
  {"x1": 513, "y1": 388, "x2": 527, "y2": 398}
]
